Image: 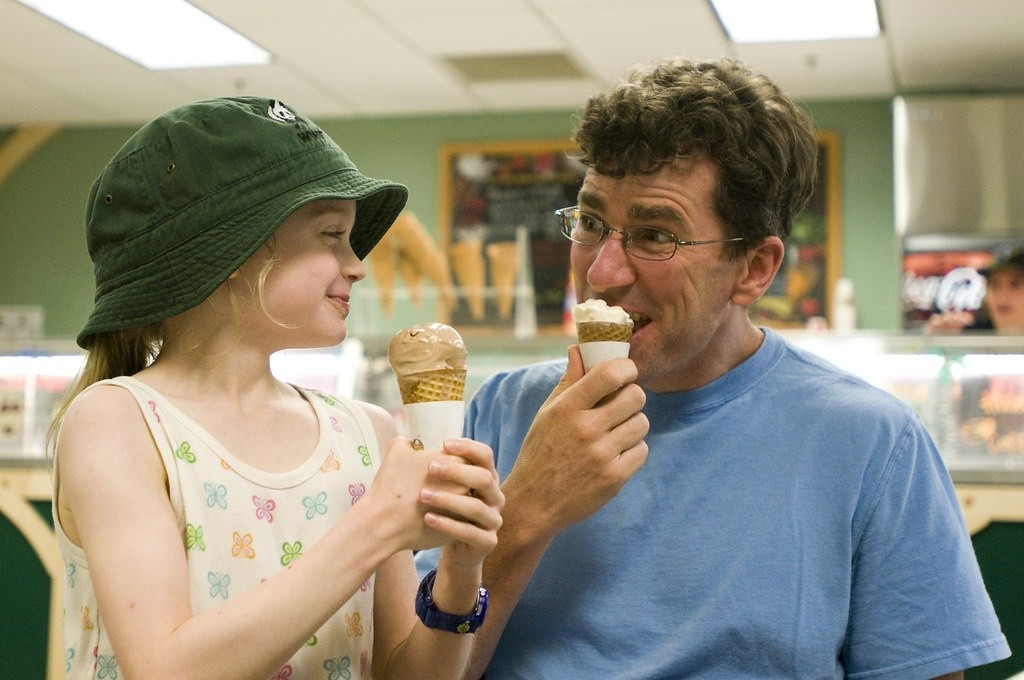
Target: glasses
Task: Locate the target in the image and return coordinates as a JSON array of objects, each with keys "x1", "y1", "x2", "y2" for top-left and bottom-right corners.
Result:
[{"x1": 554, "y1": 204, "x2": 742, "y2": 261}]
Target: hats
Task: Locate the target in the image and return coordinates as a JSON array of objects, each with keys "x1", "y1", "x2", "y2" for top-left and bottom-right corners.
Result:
[
  {"x1": 976, "y1": 244, "x2": 1024, "y2": 275},
  {"x1": 76, "y1": 96, "x2": 408, "y2": 348}
]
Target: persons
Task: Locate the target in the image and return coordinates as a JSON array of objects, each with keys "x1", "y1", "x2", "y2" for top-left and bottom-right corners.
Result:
[
  {"x1": 46, "y1": 98, "x2": 506, "y2": 680},
  {"x1": 415, "y1": 58, "x2": 1013, "y2": 680},
  {"x1": 922, "y1": 241, "x2": 1024, "y2": 454}
]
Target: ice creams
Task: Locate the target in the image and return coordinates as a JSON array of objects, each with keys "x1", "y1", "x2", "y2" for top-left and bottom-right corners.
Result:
[
  {"x1": 388, "y1": 322, "x2": 468, "y2": 451},
  {"x1": 572, "y1": 299, "x2": 634, "y2": 376}
]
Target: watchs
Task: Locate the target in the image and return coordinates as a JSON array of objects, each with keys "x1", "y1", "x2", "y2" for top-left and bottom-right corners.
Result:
[{"x1": 415, "y1": 566, "x2": 488, "y2": 634}]
